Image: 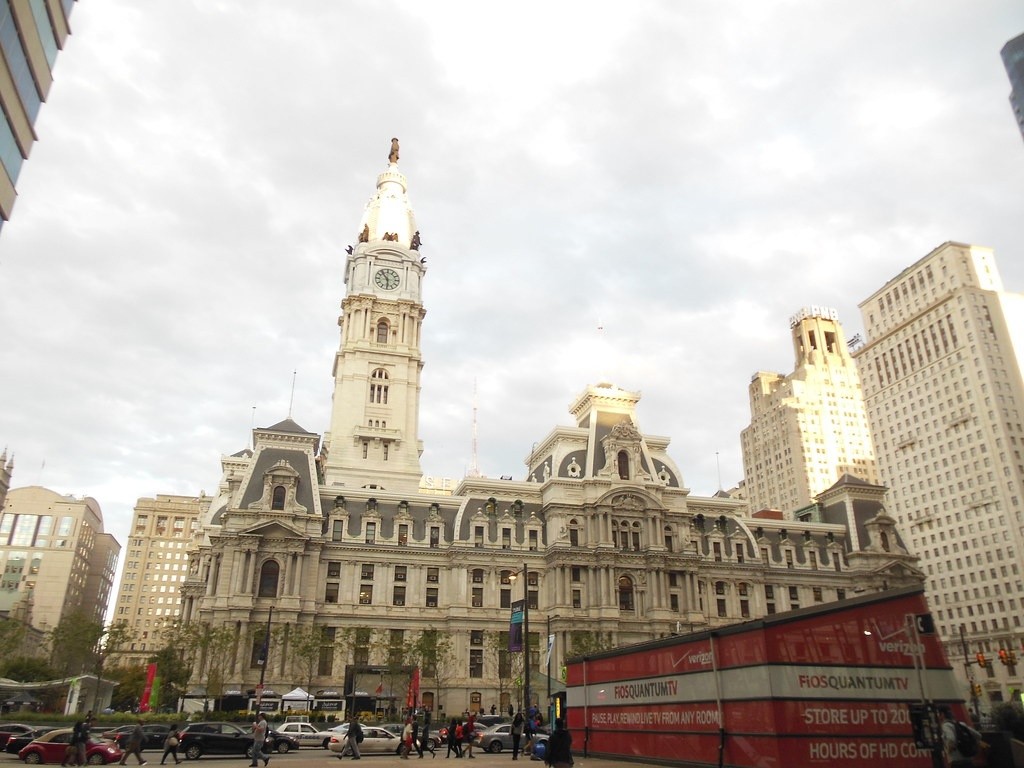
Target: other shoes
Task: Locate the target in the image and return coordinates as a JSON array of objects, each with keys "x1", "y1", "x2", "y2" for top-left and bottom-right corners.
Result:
[
  {"x1": 455, "y1": 754, "x2": 459, "y2": 758},
  {"x1": 139, "y1": 761, "x2": 148, "y2": 766},
  {"x1": 469, "y1": 754, "x2": 475, "y2": 758},
  {"x1": 249, "y1": 762, "x2": 258, "y2": 767},
  {"x1": 445, "y1": 756, "x2": 449, "y2": 758},
  {"x1": 160, "y1": 762, "x2": 167, "y2": 765},
  {"x1": 462, "y1": 750, "x2": 465, "y2": 757},
  {"x1": 512, "y1": 757, "x2": 517, "y2": 760},
  {"x1": 336, "y1": 755, "x2": 342, "y2": 760},
  {"x1": 61, "y1": 763, "x2": 68, "y2": 767},
  {"x1": 351, "y1": 756, "x2": 360, "y2": 760},
  {"x1": 119, "y1": 761, "x2": 127, "y2": 766},
  {"x1": 264, "y1": 758, "x2": 270, "y2": 766},
  {"x1": 433, "y1": 753, "x2": 436, "y2": 758},
  {"x1": 176, "y1": 760, "x2": 183, "y2": 764}
]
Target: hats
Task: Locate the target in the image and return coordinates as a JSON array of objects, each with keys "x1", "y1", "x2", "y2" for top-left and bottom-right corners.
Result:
[{"x1": 258, "y1": 713, "x2": 265, "y2": 716}]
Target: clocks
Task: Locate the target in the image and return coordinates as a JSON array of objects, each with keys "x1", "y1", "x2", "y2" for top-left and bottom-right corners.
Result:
[{"x1": 374, "y1": 268, "x2": 400, "y2": 291}]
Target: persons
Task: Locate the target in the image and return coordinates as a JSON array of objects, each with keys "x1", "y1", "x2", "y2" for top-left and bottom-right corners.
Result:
[
  {"x1": 362, "y1": 224, "x2": 369, "y2": 242},
  {"x1": 410, "y1": 231, "x2": 423, "y2": 251},
  {"x1": 334, "y1": 713, "x2": 362, "y2": 760},
  {"x1": 61, "y1": 710, "x2": 97, "y2": 767},
  {"x1": 118, "y1": 718, "x2": 149, "y2": 766},
  {"x1": 936, "y1": 703, "x2": 983, "y2": 768},
  {"x1": 479, "y1": 704, "x2": 545, "y2": 761},
  {"x1": 388, "y1": 138, "x2": 399, "y2": 163},
  {"x1": 968, "y1": 708, "x2": 991, "y2": 730},
  {"x1": 160, "y1": 724, "x2": 183, "y2": 766},
  {"x1": 544, "y1": 717, "x2": 573, "y2": 768},
  {"x1": 286, "y1": 705, "x2": 293, "y2": 716},
  {"x1": 248, "y1": 713, "x2": 270, "y2": 767},
  {"x1": 400, "y1": 714, "x2": 476, "y2": 759}
]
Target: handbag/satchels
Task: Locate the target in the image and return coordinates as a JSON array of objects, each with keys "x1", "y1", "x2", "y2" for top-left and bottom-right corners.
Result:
[
  {"x1": 469, "y1": 731, "x2": 478, "y2": 740},
  {"x1": 405, "y1": 733, "x2": 412, "y2": 747},
  {"x1": 265, "y1": 724, "x2": 269, "y2": 740},
  {"x1": 65, "y1": 746, "x2": 77, "y2": 755},
  {"x1": 168, "y1": 736, "x2": 178, "y2": 746},
  {"x1": 358, "y1": 732, "x2": 364, "y2": 743}
]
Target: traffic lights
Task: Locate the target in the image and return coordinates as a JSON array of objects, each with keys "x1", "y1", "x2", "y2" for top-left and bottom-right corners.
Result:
[
  {"x1": 975, "y1": 684, "x2": 982, "y2": 697},
  {"x1": 1010, "y1": 649, "x2": 1018, "y2": 665},
  {"x1": 999, "y1": 649, "x2": 1008, "y2": 665},
  {"x1": 976, "y1": 653, "x2": 986, "y2": 667}
]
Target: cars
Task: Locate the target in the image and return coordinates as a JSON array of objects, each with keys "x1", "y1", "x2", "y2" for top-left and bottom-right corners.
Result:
[
  {"x1": 276, "y1": 722, "x2": 339, "y2": 750},
  {"x1": 0, "y1": 723, "x2": 33, "y2": 751},
  {"x1": 377, "y1": 723, "x2": 442, "y2": 751},
  {"x1": 328, "y1": 727, "x2": 421, "y2": 756},
  {"x1": 477, "y1": 723, "x2": 550, "y2": 754},
  {"x1": 103, "y1": 724, "x2": 137, "y2": 739},
  {"x1": 241, "y1": 725, "x2": 299, "y2": 755},
  {"x1": 327, "y1": 723, "x2": 368, "y2": 733},
  {"x1": 5, "y1": 729, "x2": 59, "y2": 754},
  {"x1": 18, "y1": 729, "x2": 123, "y2": 766},
  {"x1": 115, "y1": 725, "x2": 179, "y2": 754},
  {"x1": 431, "y1": 722, "x2": 489, "y2": 744}
]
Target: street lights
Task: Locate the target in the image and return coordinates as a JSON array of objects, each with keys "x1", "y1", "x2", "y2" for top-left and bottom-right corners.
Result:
[{"x1": 508, "y1": 563, "x2": 532, "y2": 744}]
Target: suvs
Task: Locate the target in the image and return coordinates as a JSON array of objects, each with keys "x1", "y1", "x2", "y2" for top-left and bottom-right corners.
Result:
[{"x1": 179, "y1": 722, "x2": 275, "y2": 760}]
